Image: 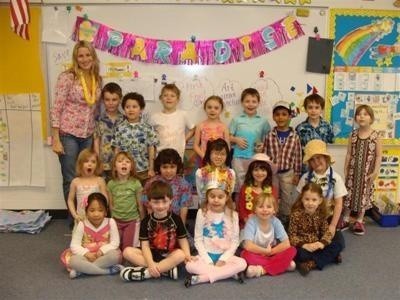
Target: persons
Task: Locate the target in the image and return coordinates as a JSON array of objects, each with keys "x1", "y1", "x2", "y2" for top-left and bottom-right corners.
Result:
[
  {"x1": 288, "y1": 183, "x2": 342, "y2": 276},
  {"x1": 66, "y1": 192, "x2": 123, "y2": 278},
  {"x1": 296, "y1": 93, "x2": 334, "y2": 177},
  {"x1": 49, "y1": 41, "x2": 104, "y2": 234},
  {"x1": 265, "y1": 100, "x2": 302, "y2": 225},
  {"x1": 120, "y1": 180, "x2": 199, "y2": 281},
  {"x1": 287, "y1": 140, "x2": 345, "y2": 251},
  {"x1": 229, "y1": 88, "x2": 271, "y2": 188},
  {"x1": 139, "y1": 147, "x2": 194, "y2": 251},
  {"x1": 111, "y1": 92, "x2": 160, "y2": 181},
  {"x1": 94, "y1": 83, "x2": 126, "y2": 179},
  {"x1": 336, "y1": 105, "x2": 381, "y2": 234},
  {"x1": 237, "y1": 153, "x2": 278, "y2": 227},
  {"x1": 193, "y1": 95, "x2": 231, "y2": 167},
  {"x1": 66, "y1": 147, "x2": 108, "y2": 237},
  {"x1": 239, "y1": 193, "x2": 297, "y2": 278},
  {"x1": 195, "y1": 138, "x2": 236, "y2": 204},
  {"x1": 185, "y1": 181, "x2": 248, "y2": 287},
  {"x1": 106, "y1": 151, "x2": 144, "y2": 250},
  {"x1": 147, "y1": 84, "x2": 196, "y2": 164}
]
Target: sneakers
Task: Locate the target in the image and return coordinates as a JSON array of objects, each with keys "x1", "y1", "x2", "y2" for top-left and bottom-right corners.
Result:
[
  {"x1": 68, "y1": 268, "x2": 81, "y2": 279},
  {"x1": 336, "y1": 219, "x2": 350, "y2": 231},
  {"x1": 353, "y1": 221, "x2": 365, "y2": 235},
  {"x1": 286, "y1": 260, "x2": 296, "y2": 272},
  {"x1": 109, "y1": 264, "x2": 125, "y2": 275},
  {"x1": 120, "y1": 266, "x2": 147, "y2": 282},
  {"x1": 245, "y1": 265, "x2": 265, "y2": 279},
  {"x1": 161, "y1": 267, "x2": 179, "y2": 280},
  {"x1": 297, "y1": 258, "x2": 315, "y2": 276},
  {"x1": 333, "y1": 253, "x2": 343, "y2": 264}
]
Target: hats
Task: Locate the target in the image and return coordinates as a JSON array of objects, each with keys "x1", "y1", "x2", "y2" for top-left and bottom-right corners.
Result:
[
  {"x1": 303, "y1": 139, "x2": 331, "y2": 164},
  {"x1": 247, "y1": 153, "x2": 273, "y2": 166},
  {"x1": 271, "y1": 101, "x2": 290, "y2": 110}
]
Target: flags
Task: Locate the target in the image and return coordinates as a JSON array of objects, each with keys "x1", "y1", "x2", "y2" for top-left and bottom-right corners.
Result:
[{"x1": 9, "y1": 1, "x2": 31, "y2": 40}]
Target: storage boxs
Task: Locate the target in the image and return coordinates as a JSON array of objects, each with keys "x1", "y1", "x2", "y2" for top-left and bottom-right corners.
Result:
[{"x1": 369, "y1": 207, "x2": 400, "y2": 227}]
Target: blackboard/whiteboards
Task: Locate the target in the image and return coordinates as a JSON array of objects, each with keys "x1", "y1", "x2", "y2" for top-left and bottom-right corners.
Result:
[{"x1": 41, "y1": 3, "x2": 330, "y2": 149}]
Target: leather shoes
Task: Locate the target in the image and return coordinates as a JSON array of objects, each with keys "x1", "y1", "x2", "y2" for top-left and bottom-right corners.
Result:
[
  {"x1": 185, "y1": 274, "x2": 194, "y2": 288},
  {"x1": 236, "y1": 271, "x2": 244, "y2": 284}
]
